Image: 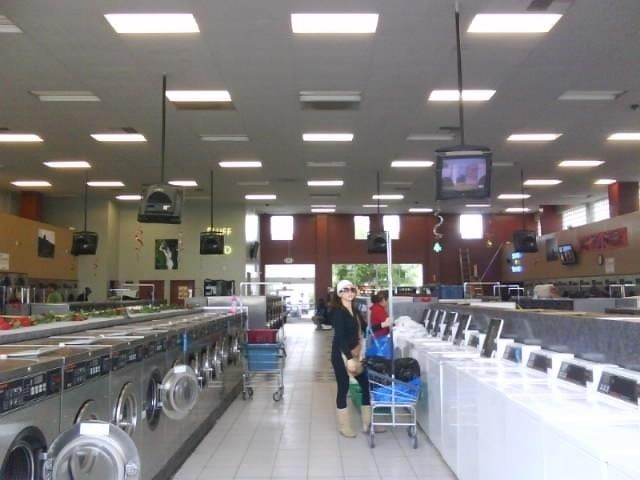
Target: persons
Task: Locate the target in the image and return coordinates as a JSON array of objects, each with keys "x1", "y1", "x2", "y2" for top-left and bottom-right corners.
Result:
[
  {"x1": 368, "y1": 289, "x2": 401, "y2": 345},
  {"x1": 311, "y1": 298, "x2": 328, "y2": 330},
  {"x1": 75, "y1": 287, "x2": 92, "y2": 302},
  {"x1": 45, "y1": 283, "x2": 63, "y2": 303},
  {"x1": 329, "y1": 279, "x2": 387, "y2": 437}
]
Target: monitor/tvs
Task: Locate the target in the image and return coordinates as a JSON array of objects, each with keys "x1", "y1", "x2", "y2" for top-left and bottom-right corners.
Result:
[
  {"x1": 70, "y1": 231, "x2": 98, "y2": 255},
  {"x1": 435, "y1": 154, "x2": 492, "y2": 199},
  {"x1": 367, "y1": 232, "x2": 387, "y2": 253},
  {"x1": 137, "y1": 183, "x2": 183, "y2": 223},
  {"x1": 199, "y1": 233, "x2": 224, "y2": 255},
  {"x1": 513, "y1": 229, "x2": 538, "y2": 253},
  {"x1": 557, "y1": 244, "x2": 577, "y2": 265},
  {"x1": 251, "y1": 241, "x2": 260, "y2": 258}
]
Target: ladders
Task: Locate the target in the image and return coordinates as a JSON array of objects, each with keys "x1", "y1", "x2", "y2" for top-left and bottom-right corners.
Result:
[{"x1": 459, "y1": 247, "x2": 473, "y2": 299}]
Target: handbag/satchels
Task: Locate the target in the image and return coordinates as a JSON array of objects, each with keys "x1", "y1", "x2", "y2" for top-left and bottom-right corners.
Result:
[{"x1": 346, "y1": 355, "x2": 364, "y2": 377}]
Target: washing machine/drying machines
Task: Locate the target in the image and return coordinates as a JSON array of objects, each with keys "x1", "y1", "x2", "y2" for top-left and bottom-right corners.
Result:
[
  {"x1": 1, "y1": 270, "x2": 288, "y2": 478},
  {"x1": 354, "y1": 273, "x2": 640, "y2": 480}
]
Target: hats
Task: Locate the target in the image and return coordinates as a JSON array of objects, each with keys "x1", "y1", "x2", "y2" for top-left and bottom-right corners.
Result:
[{"x1": 337, "y1": 279, "x2": 353, "y2": 297}]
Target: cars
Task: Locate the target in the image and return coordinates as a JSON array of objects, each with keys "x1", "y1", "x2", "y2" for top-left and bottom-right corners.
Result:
[{"x1": 285, "y1": 301, "x2": 312, "y2": 320}]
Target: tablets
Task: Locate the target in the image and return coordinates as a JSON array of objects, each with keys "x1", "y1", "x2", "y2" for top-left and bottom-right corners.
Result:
[{"x1": 420, "y1": 307, "x2": 505, "y2": 361}]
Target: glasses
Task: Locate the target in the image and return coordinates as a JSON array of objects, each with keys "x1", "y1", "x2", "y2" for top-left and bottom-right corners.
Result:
[{"x1": 340, "y1": 287, "x2": 356, "y2": 293}]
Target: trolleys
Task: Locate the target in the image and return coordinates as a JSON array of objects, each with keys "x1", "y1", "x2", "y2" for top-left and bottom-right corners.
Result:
[
  {"x1": 236, "y1": 327, "x2": 287, "y2": 403},
  {"x1": 352, "y1": 356, "x2": 422, "y2": 450}
]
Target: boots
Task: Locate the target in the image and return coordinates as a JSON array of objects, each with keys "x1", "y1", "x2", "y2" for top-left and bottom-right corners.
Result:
[
  {"x1": 336, "y1": 406, "x2": 356, "y2": 437},
  {"x1": 360, "y1": 405, "x2": 386, "y2": 433}
]
